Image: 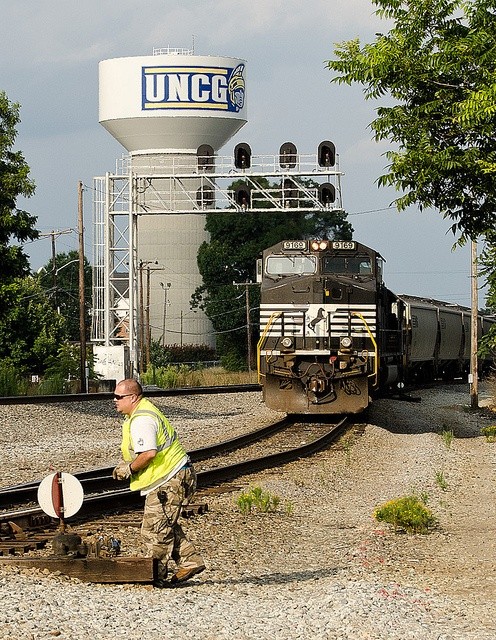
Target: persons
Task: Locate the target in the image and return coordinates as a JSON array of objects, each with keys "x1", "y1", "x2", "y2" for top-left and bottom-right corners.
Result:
[{"x1": 112, "y1": 379, "x2": 206, "y2": 586}]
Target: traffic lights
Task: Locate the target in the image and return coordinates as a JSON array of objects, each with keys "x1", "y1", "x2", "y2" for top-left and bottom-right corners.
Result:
[
  {"x1": 235, "y1": 184, "x2": 250, "y2": 204},
  {"x1": 197, "y1": 186, "x2": 212, "y2": 205},
  {"x1": 281, "y1": 180, "x2": 296, "y2": 198},
  {"x1": 235, "y1": 143, "x2": 251, "y2": 168},
  {"x1": 318, "y1": 140, "x2": 335, "y2": 166},
  {"x1": 197, "y1": 144, "x2": 213, "y2": 170},
  {"x1": 279, "y1": 142, "x2": 296, "y2": 168},
  {"x1": 318, "y1": 183, "x2": 335, "y2": 203}
]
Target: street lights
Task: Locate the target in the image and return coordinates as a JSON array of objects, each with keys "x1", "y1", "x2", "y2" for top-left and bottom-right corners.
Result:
[
  {"x1": 140, "y1": 261, "x2": 156, "y2": 375},
  {"x1": 160, "y1": 282, "x2": 171, "y2": 346}
]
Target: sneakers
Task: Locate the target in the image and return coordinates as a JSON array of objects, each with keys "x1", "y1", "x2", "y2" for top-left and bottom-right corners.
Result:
[{"x1": 171, "y1": 565, "x2": 205, "y2": 583}]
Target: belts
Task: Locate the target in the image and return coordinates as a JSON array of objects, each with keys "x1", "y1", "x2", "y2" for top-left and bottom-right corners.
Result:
[{"x1": 184, "y1": 462, "x2": 193, "y2": 467}]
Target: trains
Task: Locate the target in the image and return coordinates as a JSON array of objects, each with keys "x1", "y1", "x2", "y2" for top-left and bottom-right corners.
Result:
[{"x1": 256, "y1": 240, "x2": 496, "y2": 415}]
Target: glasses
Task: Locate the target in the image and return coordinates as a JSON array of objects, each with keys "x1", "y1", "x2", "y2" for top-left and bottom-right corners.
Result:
[{"x1": 113, "y1": 393, "x2": 137, "y2": 400}]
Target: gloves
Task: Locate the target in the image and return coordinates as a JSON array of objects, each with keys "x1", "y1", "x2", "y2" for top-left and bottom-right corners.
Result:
[{"x1": 113, "y1": 462, "x2": 133, "y2": 481}]
